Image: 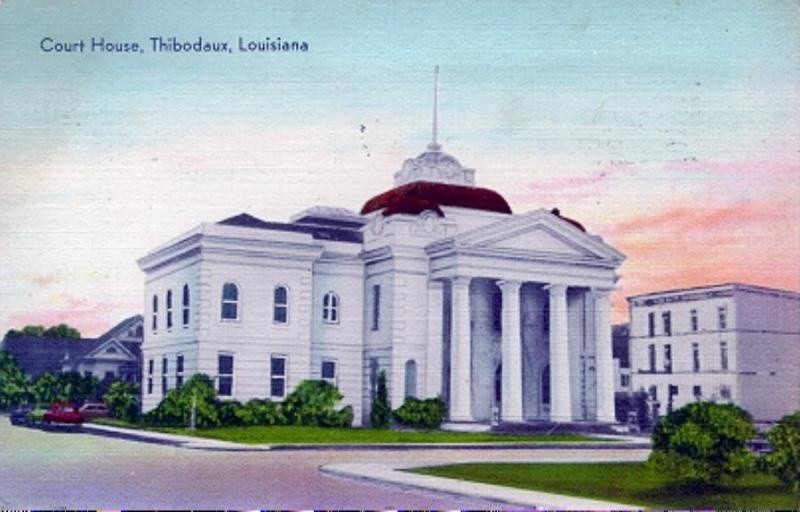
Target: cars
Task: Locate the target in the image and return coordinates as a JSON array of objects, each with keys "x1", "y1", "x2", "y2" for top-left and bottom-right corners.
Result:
[{"x1": 10, "y1": 401, "x2": 112, "y2": 428}]
